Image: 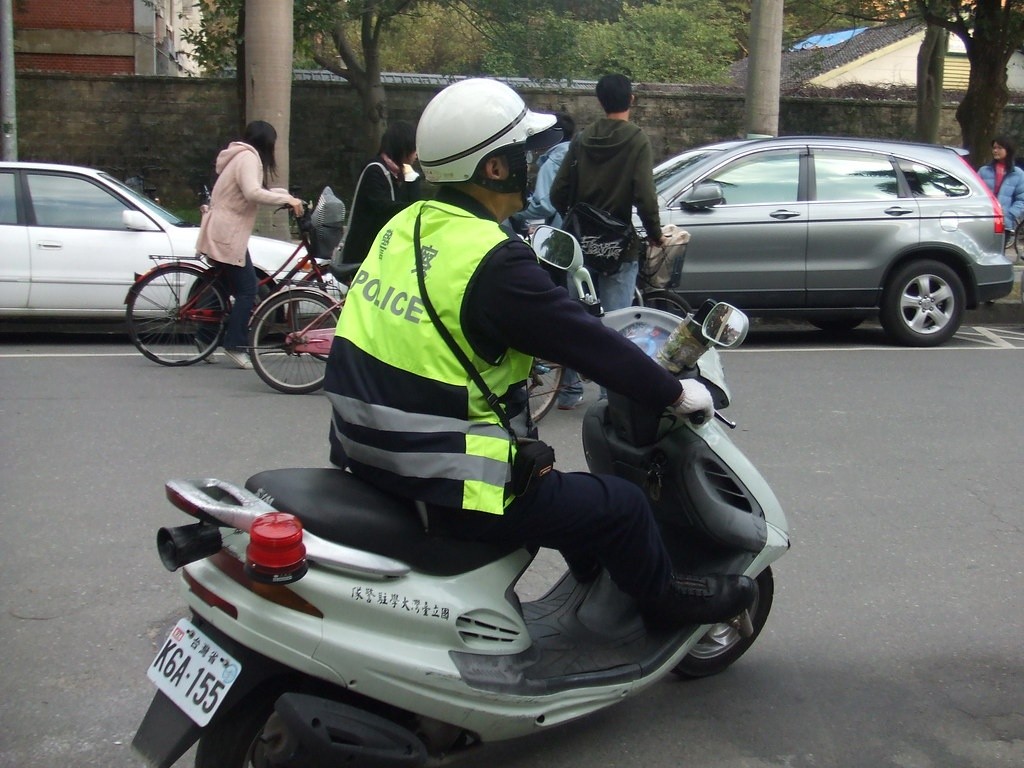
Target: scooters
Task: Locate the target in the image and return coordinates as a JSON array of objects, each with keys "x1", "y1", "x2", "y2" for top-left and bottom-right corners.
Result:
[{"x1": 126, "y1": 224, "x2": 791, "y2": 768}]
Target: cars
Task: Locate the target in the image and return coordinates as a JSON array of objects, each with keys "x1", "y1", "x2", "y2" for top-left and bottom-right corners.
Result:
[{"x1": 0, "y1": 161, "x2": 349, "y2": 335}]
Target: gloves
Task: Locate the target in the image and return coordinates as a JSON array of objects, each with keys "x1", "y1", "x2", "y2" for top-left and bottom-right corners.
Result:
[{"x1": 666, "y1": 378, "x2": 717, "y2": 429}]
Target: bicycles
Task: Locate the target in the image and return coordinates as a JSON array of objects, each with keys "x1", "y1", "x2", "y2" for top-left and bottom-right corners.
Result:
[
  {"x1": 509, "y1": 229, "x2": 690, "y2": 426},
  {"x1": 124, "y1": 198, "x2": 355, "y2": 367},
  {"x1": 234, "y1": 261, "x2": 371, "y2": 394}
]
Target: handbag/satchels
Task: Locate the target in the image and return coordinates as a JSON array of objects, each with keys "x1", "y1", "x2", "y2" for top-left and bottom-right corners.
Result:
[
  {"x1": 512, "y1": 441, "x2": 559, "y2": 503},
  {"x1": 329, "y1": 242, "x2": 363, "y2": 282},
  {"x1": 642, "y1": 229, "x2": 692, "y2": 291},
  {"x1": 557, "y1": 203, "x2": 645, "y2": 278},
  {"x1": 310, "y1": 187, "x2": 346, "y2": 225}
]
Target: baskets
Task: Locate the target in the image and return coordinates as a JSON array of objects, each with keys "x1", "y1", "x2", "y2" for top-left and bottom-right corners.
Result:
[
  {"x1": 312, "y1": 221, "x2": 351, "y2": 260},
  {"x1": 635, "y1": 227, "x2": 691, "y2": 293}
]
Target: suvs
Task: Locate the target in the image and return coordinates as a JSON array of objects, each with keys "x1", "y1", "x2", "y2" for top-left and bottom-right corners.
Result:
[{"x1": 629, "y1": 136, "x2": 1017, "y2": 347}]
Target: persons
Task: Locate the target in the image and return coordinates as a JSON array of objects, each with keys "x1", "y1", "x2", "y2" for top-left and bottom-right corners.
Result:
[
  {"x1": 326, "y1": 77, "x2": 757, "y2": 629},
  {"x1": 341, "y1": 121, "x2": 420, "y2": 281},
  {"x1": 499, "y1": 75, "x2": 667, "y2": 411},
  {"x1": 194, "y1": 120, "x2": 304, "y2": 369},
  {"x1": 976, "y1": 138, "x2": 1024, "y2": 304}
]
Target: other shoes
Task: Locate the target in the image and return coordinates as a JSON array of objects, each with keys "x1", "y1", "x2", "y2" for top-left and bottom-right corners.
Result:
[
  {"x1": 642, "y1": 574, "x2": 759, "y2": 635},
  {"x1": 194, "y1": 337, "x2": 219, "y2": 364},
  {"x1": 557, "y1": 396, "x2": 584, "y2": 410},
  {"x1": 223, "y1": 349, "x2": 267, "y2": 373},
  {"x1": 573, "y1": 550, "x2": 609, "y2": 584}
]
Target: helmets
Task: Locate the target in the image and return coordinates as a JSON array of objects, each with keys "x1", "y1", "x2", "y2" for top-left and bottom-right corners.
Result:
[{"x1": 415, "y1": 77, "x2": 559, "y2": 184}]
